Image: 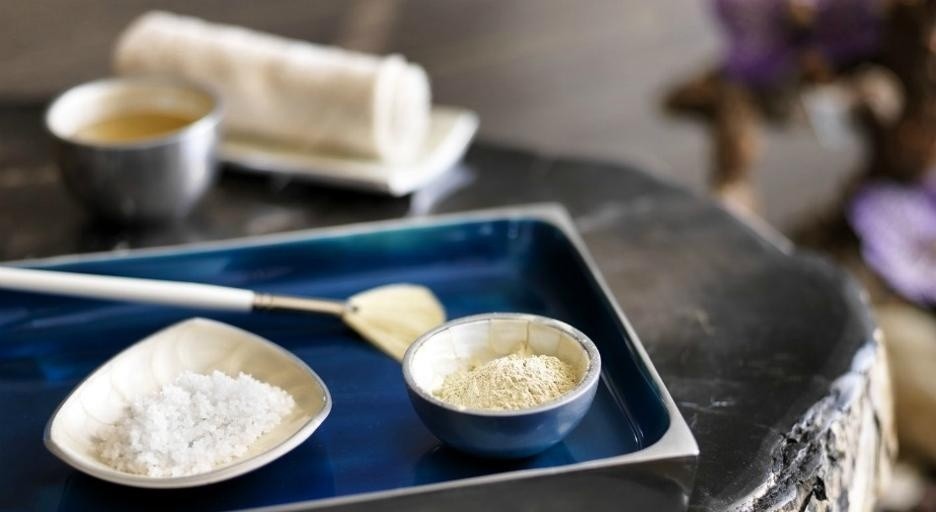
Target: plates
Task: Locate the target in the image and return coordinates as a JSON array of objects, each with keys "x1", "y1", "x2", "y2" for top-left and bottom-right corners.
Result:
[{"x1": 40, "y1": 311, "x2": 336, "y2": 494}]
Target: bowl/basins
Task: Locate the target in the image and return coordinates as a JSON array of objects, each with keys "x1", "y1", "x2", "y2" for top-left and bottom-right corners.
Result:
[
  {"x1": 42, "y1": 76, "x2": 231, "y2": 227},
  {"x1": 396, "y1": 310, "x2": 602, "y2": 459}
]
